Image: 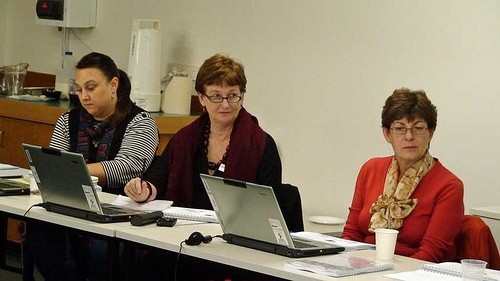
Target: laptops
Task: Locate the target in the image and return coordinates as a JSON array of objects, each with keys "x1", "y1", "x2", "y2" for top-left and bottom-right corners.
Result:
[
  {"x1": 199, "y1": 173, "x2": 345, "y2": 258},
  {"x1": 0, "y1": 178, "x2": 30, "y2": 196},
  {"x1": 21, "y1": 143, "x2": 150, "y2": 224}
]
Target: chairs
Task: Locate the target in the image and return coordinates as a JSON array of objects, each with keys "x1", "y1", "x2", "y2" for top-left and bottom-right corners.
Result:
[
  {"x1": 279, "y1": 184, "x2": 303, "y2": 232},
  {"x1": 460, "y1": 215, "x2": 500, "y2": 271}
]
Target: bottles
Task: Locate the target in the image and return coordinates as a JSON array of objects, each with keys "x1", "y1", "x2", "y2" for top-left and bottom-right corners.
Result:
[
  {"x1": 90, "y1": 175, "x2": 103, "y2": 203},
  {"x1": 54, "y1": 52, "x2": 76, "y2": 98},
  {"x1": 29, "y1": 170, "x2": 39, "y2": 192}
]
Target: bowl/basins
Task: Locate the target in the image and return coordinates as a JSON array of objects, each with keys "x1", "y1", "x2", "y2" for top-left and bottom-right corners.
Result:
[
  {"x1": 42, "y1": 89, "x2": 62, "y2": 103},
  {"x1": 69, "y1": 93, "x2": 80, "y2": 106}
]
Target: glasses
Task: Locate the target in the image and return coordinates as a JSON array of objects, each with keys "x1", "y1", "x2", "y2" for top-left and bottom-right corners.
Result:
[
  {"x1": 201, "y1": 92, "x2": 244, "y2": 103},
  {"x1": 389, "y1": 127, "x2": 428, "y2": 135}
]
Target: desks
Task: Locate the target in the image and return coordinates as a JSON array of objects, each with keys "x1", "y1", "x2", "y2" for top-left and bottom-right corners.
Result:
[
  {"x1": 0, "y1": 164, "x2": 500, "y2": 281},
  {"x1": 469, "y1": 206, "x2": 500, "y2": 258},
  {"x1": 0, "y1": 95, "x2": 202, "y2": 168}
]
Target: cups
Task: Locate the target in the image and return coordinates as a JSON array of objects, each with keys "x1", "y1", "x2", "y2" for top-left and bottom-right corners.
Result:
[
  {"x1": 460, "y1": 258, "x2": 487, "y2": 281},
  {"x1": 374, "y1": 228, "x2": 399, "y2": 261}
]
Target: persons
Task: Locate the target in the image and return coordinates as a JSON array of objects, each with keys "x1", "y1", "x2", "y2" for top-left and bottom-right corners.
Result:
[
  {"x1": 123, "y1": 53, "x2": 283, "y2": 211},
  {"x1": 48, "y1": 52, "x2": 159, "y2": 197},
  {"x1": 340, "y1": 88, "x2": 464, "y2": 263}
]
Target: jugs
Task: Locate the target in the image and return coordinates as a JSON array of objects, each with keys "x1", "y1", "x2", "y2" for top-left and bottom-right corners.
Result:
[{"x1": 0, "y1": 62, "x2": 29, "y2": 95}]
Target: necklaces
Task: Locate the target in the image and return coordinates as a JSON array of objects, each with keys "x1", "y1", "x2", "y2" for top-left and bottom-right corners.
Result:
[{"x1": 203, "y1": 123, "x2": 230, "y2": 170}]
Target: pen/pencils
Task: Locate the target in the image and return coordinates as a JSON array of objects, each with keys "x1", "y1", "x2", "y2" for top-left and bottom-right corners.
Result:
[{"x1": 138, "y1": 158, "x2": 147, "y2": 195}]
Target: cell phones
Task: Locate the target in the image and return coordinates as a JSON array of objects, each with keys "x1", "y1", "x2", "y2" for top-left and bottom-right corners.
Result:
[{"x1": 157, "y1": 218, "x2": 178, "y2": 227}]
